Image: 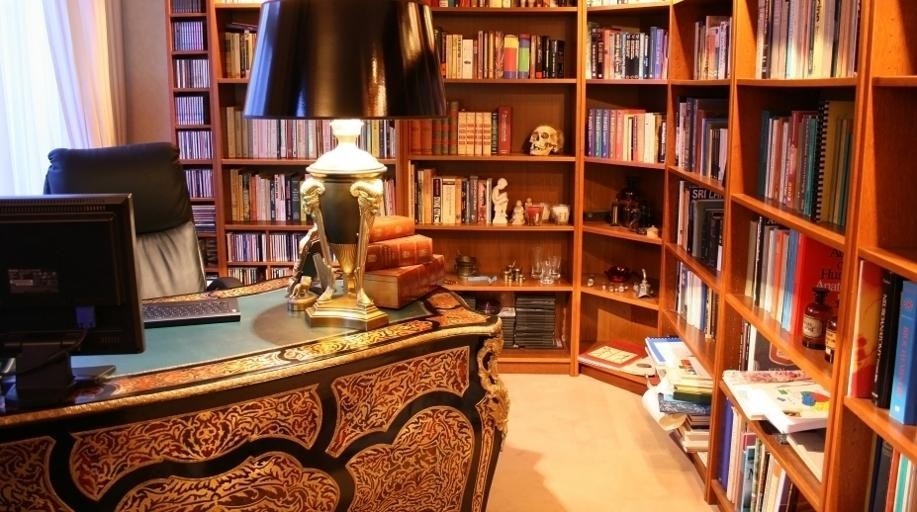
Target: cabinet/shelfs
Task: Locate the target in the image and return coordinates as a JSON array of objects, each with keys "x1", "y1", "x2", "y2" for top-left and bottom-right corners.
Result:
[
  {"x1": 162, "y1": 0, "x2": 218, "y2": 273},
  {"x1": 213, "y1": 0, "x2": 581, "y2": 378},
  {"x1": 575, "y1": 0, "x2": 916, "y2": 511}
]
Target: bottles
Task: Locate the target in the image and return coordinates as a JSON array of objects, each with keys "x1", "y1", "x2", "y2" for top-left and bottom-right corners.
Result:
[
  {"x1": 802, "y1": 286, "x2": 841, "y2": 361},
  {"x1": 457, "y1": 256, "x2": 472, "y2": 278},
  {"x1": 611, "y1": 176, "x2": 653, "y2": 233}
]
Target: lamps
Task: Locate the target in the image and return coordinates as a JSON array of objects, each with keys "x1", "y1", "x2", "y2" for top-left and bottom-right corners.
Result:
[{"x1": 240, "y1": 0, "x2": 448, "y2": 331}]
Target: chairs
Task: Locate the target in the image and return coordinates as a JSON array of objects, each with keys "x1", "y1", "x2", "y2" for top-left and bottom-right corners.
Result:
[{"x1": 46, "y1": 139, "x2": 242, "y2": 300}]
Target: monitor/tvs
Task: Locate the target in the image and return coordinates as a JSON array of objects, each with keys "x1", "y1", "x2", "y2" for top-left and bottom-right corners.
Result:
[{"x1": 0, "y1": 192, "x2": 147, "y2": 408}]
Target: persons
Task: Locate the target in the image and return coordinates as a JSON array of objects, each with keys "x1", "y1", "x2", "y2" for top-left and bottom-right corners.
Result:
[
  {"x1": 493, "y1": 177, "x2": 509, "y2": 217},
  {"x1": 512, "y1": 200, "x2": 525, "y2": 220}
]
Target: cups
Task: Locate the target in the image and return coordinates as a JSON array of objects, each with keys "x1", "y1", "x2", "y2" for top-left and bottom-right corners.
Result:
[
  {"x1": 524, "y1": 203, "x2": 544, "y2": 226},
  {"x1": 530, "y1": 248, "x2": 561, "y2": 286},
  {"x1": 551, "y1": 204, "x2": 570, "y2": 226}
]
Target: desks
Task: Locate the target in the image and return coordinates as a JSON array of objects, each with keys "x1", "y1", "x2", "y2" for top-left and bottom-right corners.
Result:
[{"x1": 0, "y1": 274, "x2": 510, "y2": 511}]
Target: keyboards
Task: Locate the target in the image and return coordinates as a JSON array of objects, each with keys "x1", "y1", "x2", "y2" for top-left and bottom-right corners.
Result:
[{"x1": 142, "y1": 297, "x2": 241, "y2": 326}]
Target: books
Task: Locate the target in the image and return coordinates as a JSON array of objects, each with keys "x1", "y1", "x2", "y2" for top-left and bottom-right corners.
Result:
[
  {"x1": 222, "y1": 21, "x2": 395, "y2": 287},
  {"x1": 462, "y1": 294, "x2": 562, "y2": 349},
  {"x1": 589, "y1": 23, "x2": 666, "y2": 166},
  {"x1": 579, "y1": 336, "x2": 713, "y2": 455},
  {"x1": 408, "y1": 0, "x2": 575, "y2": 153},
  {"x1": 717, "y1": 1, "x2": 917, "y2": 512},
  {"x1": 673, "y1": 13, "x2": 733, "y2": 337},
  {"x1": 355, "y1": 216, "x2": 444, "y2": 309},
  {"x1": 410, "y1": 165, "x2": 493, "y2": 224},
  {"x1": 171, "y1": 1, "x2": 217, "y2": 270}
]
